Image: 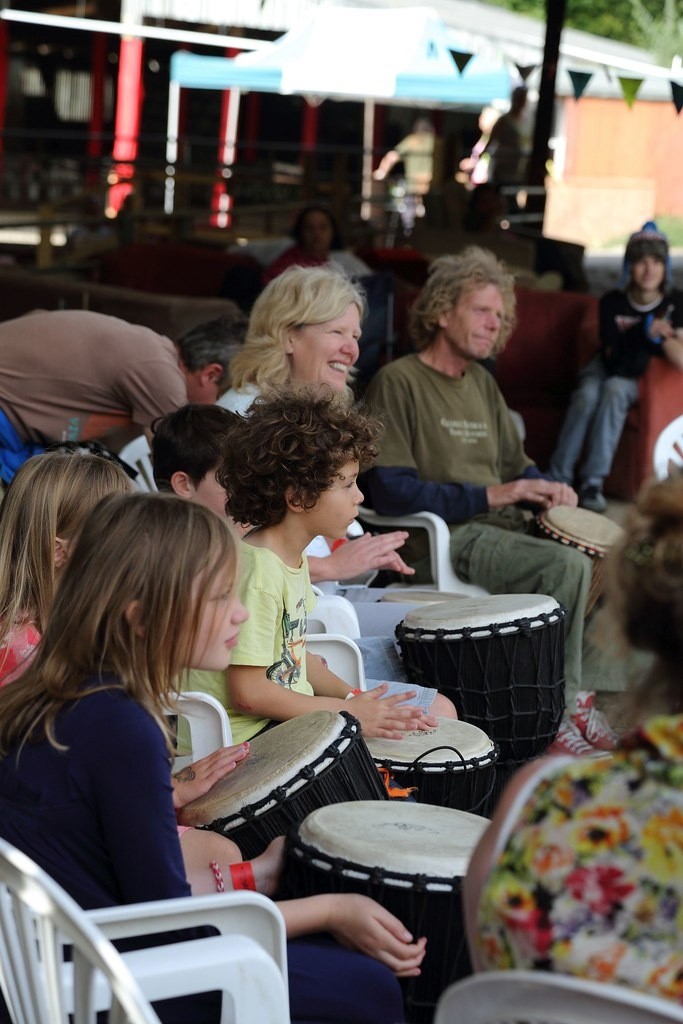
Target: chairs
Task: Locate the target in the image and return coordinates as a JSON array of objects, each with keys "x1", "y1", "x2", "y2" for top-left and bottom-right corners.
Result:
[{"x1": 0, "y1": 438, "x2": 683, "y2": 1024}]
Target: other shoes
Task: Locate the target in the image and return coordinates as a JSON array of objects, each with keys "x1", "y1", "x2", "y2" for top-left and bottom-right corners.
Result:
[{"x1": 580, "y1": 483, "x2": 607, "y2": 512}]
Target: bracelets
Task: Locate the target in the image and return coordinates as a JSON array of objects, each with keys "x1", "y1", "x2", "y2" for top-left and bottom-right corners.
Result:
[
  {"x1": 345, "y1": 689, "x2": 362, "y2": 701},
  {"x1": 209, "y1": 861, "x2": 225, "y2": 892},
  {"x1": 331, "y1": 539, "x2": 346, "y2": 553},
  {"x1": 660, "y1": 332, "x2": 679, "y2": 341},
  {"x1": 229, "y1": 862, "x2": 257, "y2": 891}
]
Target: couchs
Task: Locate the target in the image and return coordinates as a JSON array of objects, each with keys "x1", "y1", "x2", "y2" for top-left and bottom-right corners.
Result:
[{"x1": 0, "y1": 248, "x2": 683, "y2": 498}]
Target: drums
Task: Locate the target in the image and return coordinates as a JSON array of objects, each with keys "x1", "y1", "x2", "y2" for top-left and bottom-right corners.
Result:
[
  {"x1": 394, "y1": 593, "x2": 564, "y2": 791},
  {"x1": 527, "y1": 505, "x2": 629, "y2": 622},
  {"x1": 175, "y1": 707, "x2": 389, "y2": 891},
  {"x1": 360, "y1": 714, "x2": 499, "y2": 815},
  {"x1": 289, "y1": 797, "x2": 496, "y2": 1024}
]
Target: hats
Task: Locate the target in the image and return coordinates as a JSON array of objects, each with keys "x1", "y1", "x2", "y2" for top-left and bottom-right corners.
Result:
[{"x1": 617, "y1": 221, "x2": 673, "y2": 294}]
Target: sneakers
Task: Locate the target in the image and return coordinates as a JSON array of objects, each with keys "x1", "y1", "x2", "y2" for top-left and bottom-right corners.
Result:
[
  {"x1": 570, "y1": 692, "x2": 622, "y2": 750},
  {"x1": 547, "y1": 721, "x2": 614, "y2": 760}
]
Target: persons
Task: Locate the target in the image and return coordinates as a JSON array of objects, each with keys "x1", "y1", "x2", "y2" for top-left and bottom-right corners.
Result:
[
  {"x1": 0, "y1": 491, "x2": 428, "y2": 1024},
  {"x1": 463, "y1": 466, "x2": 683, "y2": 1006},
  {"x1": 374, "y1": 87, "x2": 533, "y2": 223},
  {"x1": 0, "y1": 388, "x2": 457, "y2": 806},
  {"x1": 0, "y1": 184, "x2": 683, "y2": 761}
]
